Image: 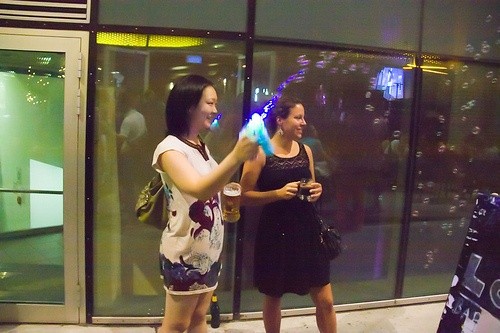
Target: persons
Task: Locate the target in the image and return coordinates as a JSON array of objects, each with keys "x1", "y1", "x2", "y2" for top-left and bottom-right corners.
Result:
[
  {"x1": 114, "y1": 90, "x2": 500, "y2": 224},
  {"x1": 241, "y1": 97, "x2": 337, "y2": 333},
  {"x1": 151, "y1": 74, "x2": 259, "y2": 333}
]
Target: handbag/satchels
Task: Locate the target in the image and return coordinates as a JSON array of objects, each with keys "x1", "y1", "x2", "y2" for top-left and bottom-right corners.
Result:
[
  {"x1": 135, "y1": 172, "x2": 169, "y2": 230},
  {"x1": 317, "y1": 223, "x2": 342, "y2": 259}
]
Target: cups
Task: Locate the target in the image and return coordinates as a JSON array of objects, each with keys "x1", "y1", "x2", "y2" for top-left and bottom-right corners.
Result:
[
  {"x1": 296, "y1": 177, "x2": 314, "y2": 201},
  {"x1": 221, "y1": 182, "x2": 240, "y2": 223}
]
